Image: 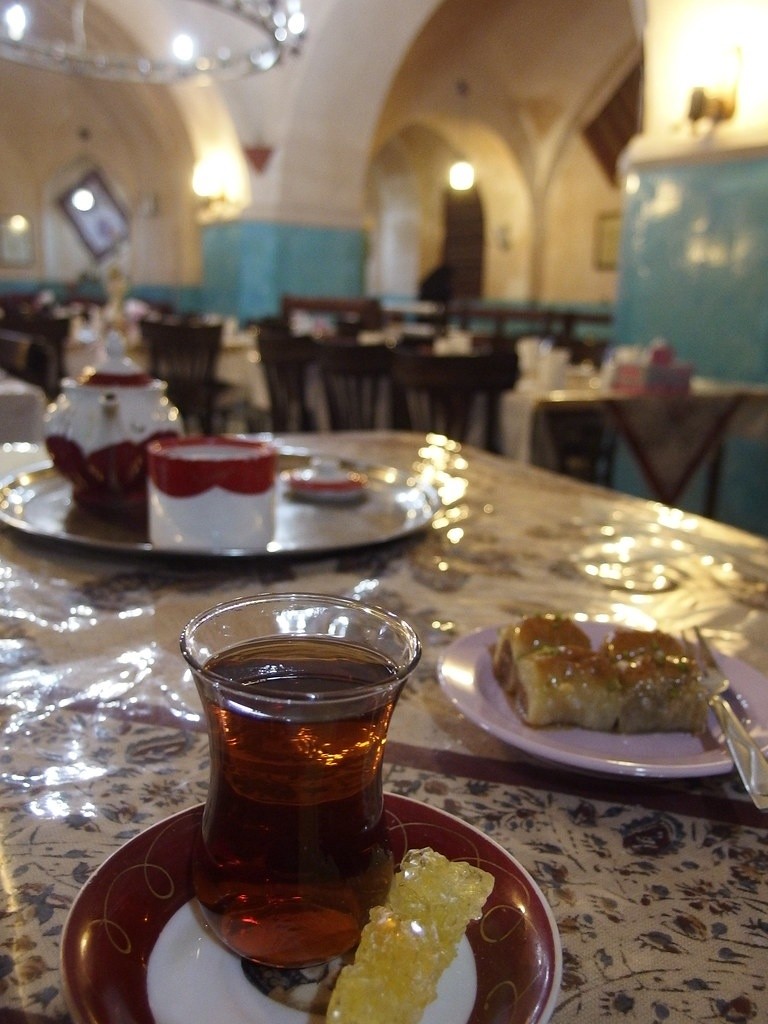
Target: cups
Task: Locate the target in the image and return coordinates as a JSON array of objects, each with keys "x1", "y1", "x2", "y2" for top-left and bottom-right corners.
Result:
[
  {"x1": 180, "y1": 596, "x2": 425, "y2": 967},
  {"x1": 146, "y1": 434, "x2": 277, "y2": 549}
]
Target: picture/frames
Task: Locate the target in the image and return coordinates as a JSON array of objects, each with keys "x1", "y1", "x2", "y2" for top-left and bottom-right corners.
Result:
[{"x1": 56, "y1": 170, "x2": 130, "y2": 260}]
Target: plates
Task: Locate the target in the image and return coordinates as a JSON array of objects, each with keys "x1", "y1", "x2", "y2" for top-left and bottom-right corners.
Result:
[
  {"x1": 57, "y1": 789, "x2": 564, "y2": 1024},
  {"x1": 439, "y1": 621, "x2": 768, "y2": 782}
]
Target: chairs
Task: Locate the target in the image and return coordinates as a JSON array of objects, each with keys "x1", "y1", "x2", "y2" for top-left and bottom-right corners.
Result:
[{"x1": 6, "y1": 293, "x2": 613, "y2": 457}]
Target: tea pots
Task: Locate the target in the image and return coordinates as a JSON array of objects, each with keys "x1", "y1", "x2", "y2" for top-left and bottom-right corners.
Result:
[{"x1": 42, "y1": 327, "x2": 185, "y2": 513}]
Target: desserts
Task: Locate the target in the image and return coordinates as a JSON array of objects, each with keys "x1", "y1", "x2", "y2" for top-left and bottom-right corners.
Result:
[{"x1": 492, "y1": 609, "x2": 710, "y2": 735}]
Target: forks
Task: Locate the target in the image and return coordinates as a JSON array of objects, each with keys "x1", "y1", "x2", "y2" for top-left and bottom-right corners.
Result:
[{"x1": 680, "y1": 625, "x2": 768, "y2": 812}]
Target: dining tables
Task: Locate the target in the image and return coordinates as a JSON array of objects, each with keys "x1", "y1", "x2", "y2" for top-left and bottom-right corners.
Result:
[{"x1": 0, "y1": 430, "x2": 768, "y2": 1023}]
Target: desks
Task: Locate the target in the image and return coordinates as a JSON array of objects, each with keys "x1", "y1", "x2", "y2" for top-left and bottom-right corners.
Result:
[{"x1": 509, "y1": 379, "x2": 768, "y2": 517}]
